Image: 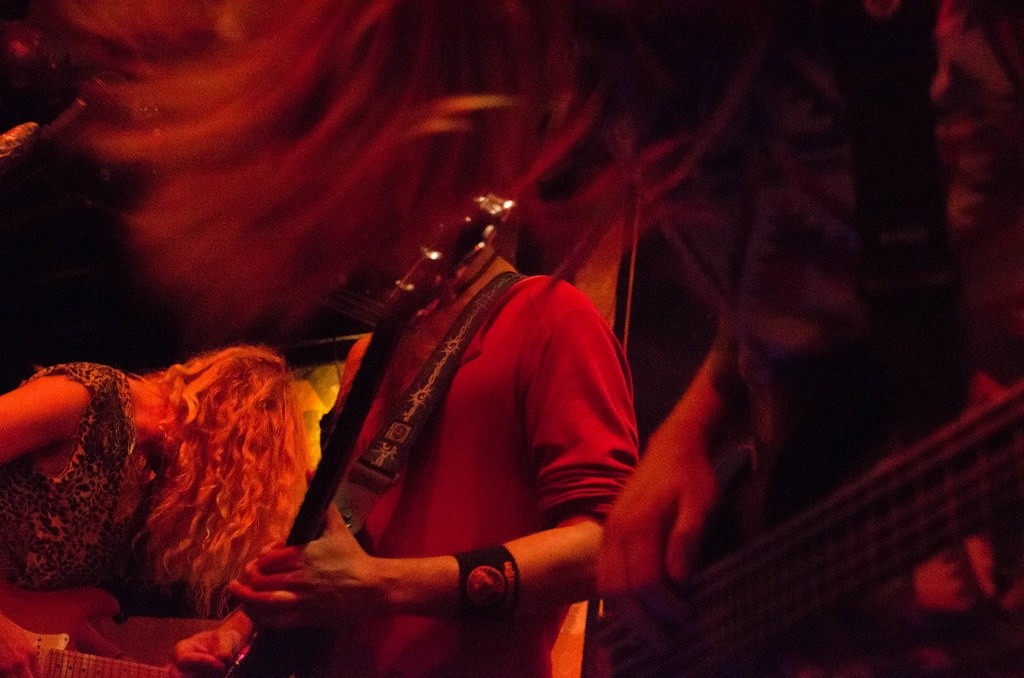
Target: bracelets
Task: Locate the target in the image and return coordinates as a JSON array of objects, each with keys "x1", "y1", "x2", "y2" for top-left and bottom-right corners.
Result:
[{"x1": 456, "y1": 546, "x2": 520, "y2": 618}]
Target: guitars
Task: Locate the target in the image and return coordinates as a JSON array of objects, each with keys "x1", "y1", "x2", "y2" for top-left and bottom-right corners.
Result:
[
  {"x1": 0, "y1": 611, "x2": 184, "y2": 675},
  {"x1": 215, "y1": 189, "x2": 517, "y2": 677}
]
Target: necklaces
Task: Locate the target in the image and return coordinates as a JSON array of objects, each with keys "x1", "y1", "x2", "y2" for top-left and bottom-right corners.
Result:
[{"x1": 414, "y1": 249, "x2": 499, "y2": 327}]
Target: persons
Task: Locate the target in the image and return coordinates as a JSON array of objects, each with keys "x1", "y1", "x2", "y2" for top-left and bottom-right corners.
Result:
[
  {"x1": 0, "y1": 344, "x2": 306, "y2": 678},
  {"x1": 171, "y1": 226, "x2": 639, "y2": 678},
  {"x1": 37, "y1": 2, "x2": 1024, "y2": 678}
]
